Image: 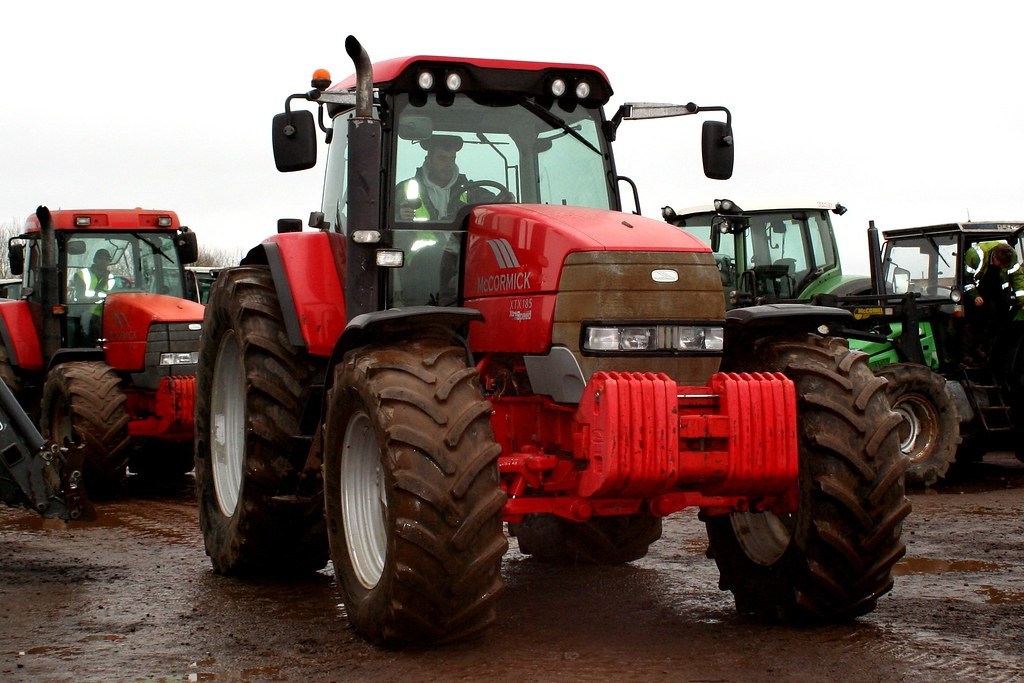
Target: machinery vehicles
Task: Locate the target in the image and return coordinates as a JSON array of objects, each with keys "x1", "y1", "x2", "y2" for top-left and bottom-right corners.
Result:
[
  {"x1": 0, "y1": 206, "x2": 223, "y2": 502},
  {"x1": 661, "y1": 199, "x2": 1024, "y2": 495},
  {"x1": 193, "y1": 36, "x2": 913, "y2": 652}
]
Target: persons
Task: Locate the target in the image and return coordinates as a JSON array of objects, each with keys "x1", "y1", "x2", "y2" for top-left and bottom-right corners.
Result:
[
  {"x1": 963, "y1": 240, "x2": 1024, "y2": 349},
  {"x1": 67, "y1": 248, "x2": 114, "y2": 348},
  {"x1": 395, "y1": 135, "x2": 515, "y2": 308}
]
